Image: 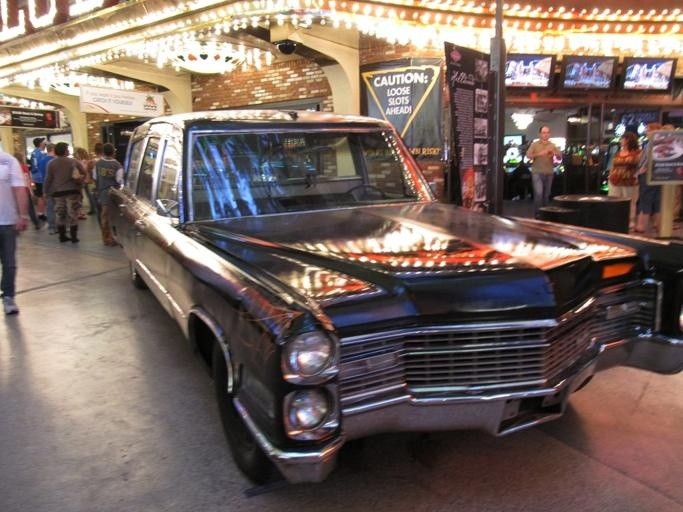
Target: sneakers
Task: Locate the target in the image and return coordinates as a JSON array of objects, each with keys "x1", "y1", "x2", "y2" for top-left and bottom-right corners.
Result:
[
  {"x1": 35, "y1": 211, "x2": 93, "y2": 243},
  {"x1": 3, "y1": 296, "x2": 18, "y2": 314}
]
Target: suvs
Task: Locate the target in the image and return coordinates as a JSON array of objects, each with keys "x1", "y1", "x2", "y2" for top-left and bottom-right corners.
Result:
[{"x1": 105, "y1": 107, "x2": 683, "y2": 485}]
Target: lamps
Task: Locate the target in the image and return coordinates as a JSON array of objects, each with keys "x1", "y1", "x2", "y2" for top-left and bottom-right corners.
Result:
[{"x1": 168, "y1": 34, "x2": 248, "y2": 74}]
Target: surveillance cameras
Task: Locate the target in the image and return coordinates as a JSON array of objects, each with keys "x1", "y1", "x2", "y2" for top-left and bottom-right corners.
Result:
[{"x1": 276, "y1": 40, "x2": 296, "y2": 55}]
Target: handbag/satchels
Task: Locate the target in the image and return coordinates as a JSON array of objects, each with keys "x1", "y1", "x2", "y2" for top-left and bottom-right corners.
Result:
[{"x1": 71, "y1": 166, "x2": 81, "y2": 179}]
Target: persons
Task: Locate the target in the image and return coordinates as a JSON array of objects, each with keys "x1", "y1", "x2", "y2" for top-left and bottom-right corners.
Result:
[
  {"x1": 526, "y1": 125, "x2": 563, "y2": 215},
  {"x1": 606, "y1": 120, "x2": 683, "y2": 233},
  {"x1": 0, "y1": 143, "x2": 32, "y2": 315},
  {"x1": 14, "y1": 134, "x2": 126, "y2": 249}
]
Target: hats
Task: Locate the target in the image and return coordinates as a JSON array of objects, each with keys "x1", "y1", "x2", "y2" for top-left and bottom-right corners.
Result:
[{"x1": 34, "y1": 138, "x2": 45, "y2": 147}]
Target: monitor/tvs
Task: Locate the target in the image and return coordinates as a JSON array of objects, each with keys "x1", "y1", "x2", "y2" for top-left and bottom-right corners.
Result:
[
  {"x1": 504, "y1": 53, "x2": 557, "y2": 90},
  {"x1": 621, "y1": 56, "x2": 678, "y2": 94},
  {"x1": 503, "y1": 134, "x2": 523, "y2": 147},
  {"x1": 560, "y1": 54, "x2": 619, "y2": 91}
]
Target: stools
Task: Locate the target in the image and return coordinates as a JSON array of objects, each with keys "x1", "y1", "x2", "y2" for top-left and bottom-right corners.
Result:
[
  {"x1": 555, "y1": 173, "x2": 576, "y2": 195},
  {"x1": 519, "y1": 173, "x2": 531, "y2": 201}
]
